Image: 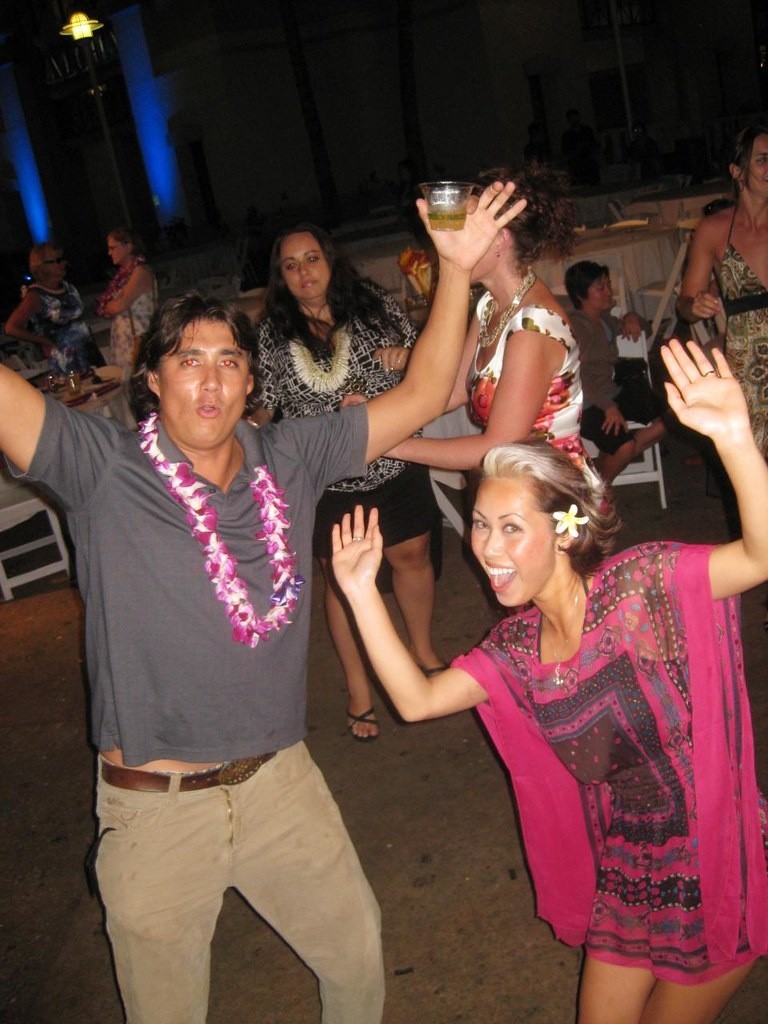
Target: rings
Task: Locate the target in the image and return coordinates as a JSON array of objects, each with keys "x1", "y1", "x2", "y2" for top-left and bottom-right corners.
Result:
[
  {"x1": 704, "y1": 371, "x2": 714, "y2": 377},
  {"x1": 353, "y1": 537, "x2": 362, "y2": 541}
]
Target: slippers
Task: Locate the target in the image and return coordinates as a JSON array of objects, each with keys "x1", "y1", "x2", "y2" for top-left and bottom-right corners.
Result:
[
  {"x1": 346, "y1": 706, "x2": 381, "y2": 742},
  {"x1": 420, "y1": 662, "x2": 451, "y2": 679}
]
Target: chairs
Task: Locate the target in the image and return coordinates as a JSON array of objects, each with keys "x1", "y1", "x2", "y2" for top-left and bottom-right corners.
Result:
[{"x1": 578, "y1": 329, "x2": 667, "y2": 509}]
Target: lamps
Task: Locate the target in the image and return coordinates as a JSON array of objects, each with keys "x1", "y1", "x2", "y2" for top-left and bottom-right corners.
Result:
[{"x1": 60, "y1": 0, "x2": 104, "y2": 40}]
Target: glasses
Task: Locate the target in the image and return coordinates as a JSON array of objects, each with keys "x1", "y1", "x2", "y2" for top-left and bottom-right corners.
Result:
[
  {"x1": 41, "y1": 256, "x2": 63, "y2": 265},
  {"x1": 107, "y1": 245, "x2": 120, "y2": 250}
]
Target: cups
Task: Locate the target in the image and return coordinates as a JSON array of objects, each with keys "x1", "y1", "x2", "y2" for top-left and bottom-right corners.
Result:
[
  {"x1": 43, "y1": 369, "x2": 80, "y2": 394},
  {"x1": 418, "y1": 180, "x2": 475, "y2": 232}
]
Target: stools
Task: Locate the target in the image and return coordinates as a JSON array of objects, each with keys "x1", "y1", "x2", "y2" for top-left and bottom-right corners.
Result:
[{"x1": 0, "y1": 464, "x2": 75, "y2": 600}]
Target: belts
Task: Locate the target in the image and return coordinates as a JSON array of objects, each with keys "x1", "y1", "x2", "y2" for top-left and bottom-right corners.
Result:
[{"x1": 100, "y1": 751, "x2": 278, "y2": 793}]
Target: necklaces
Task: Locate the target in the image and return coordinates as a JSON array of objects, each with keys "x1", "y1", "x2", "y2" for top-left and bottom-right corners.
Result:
[
  {"x1": 479, "y1": 270, "x2": 537, "y2": 347},
  {"x1": 289, "y1": 324, "x2": 351, "y2": 394},
  {"x1": 545, "y1": 588, "x2": 579, "y2": 685},
  {"x1": 97, "y1": 255, "x2": 145, "y2": 316},
  {"x1": 137, "y1": 412, "x2": 305, "y2": 647}
]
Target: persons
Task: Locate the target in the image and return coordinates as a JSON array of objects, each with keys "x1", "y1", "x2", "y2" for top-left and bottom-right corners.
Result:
[
  {"x1": 0, "y1": 181, "x2": 527, "y2": 1024},
  {"x1": 677, "y1": 122, "x2": 768, "y2": 451},
  {"x1": 340, "y1": 159, "x2": 608, "y2": 515},
  {"x1": 332, "y1": 339, "x2": 768, "y2": 1024},
  {"x1": 6, "y1": 243, "x2": 107, "y2": 380},
  {"x1": 564, "y1": 260, "x2": 667, "y2": 484},
  {"x1": 104, "y1": 228, "x2": 158, "y2": 369},
  {"x1": 246, "y1": 223, "x2": 446, "y2": 741}
]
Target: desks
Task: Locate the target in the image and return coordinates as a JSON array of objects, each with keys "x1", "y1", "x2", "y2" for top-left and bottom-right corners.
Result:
[
  {"x1": 41, "y1": 364, "x2": 137, "y2": 433},
  {"x1": 523, "y1": 221, "x2": 683, "y2": 322}
]
